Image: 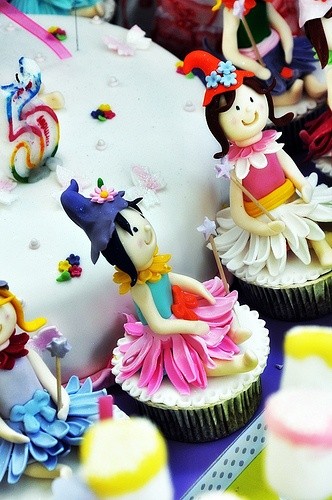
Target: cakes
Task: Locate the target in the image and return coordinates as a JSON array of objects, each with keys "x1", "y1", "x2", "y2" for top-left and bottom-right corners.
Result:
[{"x1": 0, "y1": 0, "x2": 332, "y2": 500}]
